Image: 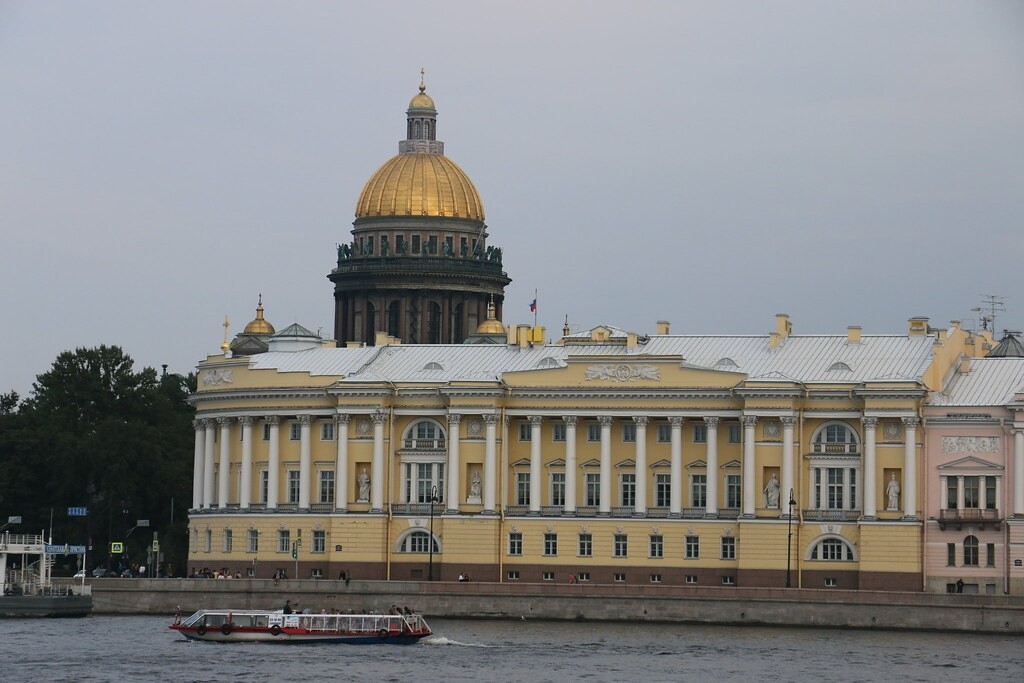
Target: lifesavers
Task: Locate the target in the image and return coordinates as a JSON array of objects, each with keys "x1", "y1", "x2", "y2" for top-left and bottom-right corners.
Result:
[
  {"x1": 270, "y1": 624, "x2": 282, "y2": 636},
  {"x1": 378, "y1": 628, "x2": 389, "y2": 640},
  {"x1": 221, "y1": 623, "x2": 231, "y2": 636},
  {"x1": 195, "y1": 624, "x2": 208, "y2": 635}
]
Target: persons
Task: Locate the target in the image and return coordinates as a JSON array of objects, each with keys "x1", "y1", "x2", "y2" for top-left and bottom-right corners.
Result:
[
  {"x1": 764, "y1": 473, "x2": 780, "y2": 505},
  {"x1": 339, "y1": 569, "x2": 352, "y2": 586},
  {"x1": 569, "y1": 573, "x2": 575, "y2": 584},
  {"x1": 358, "y1": 468, "x2": 370, "y2": 500},
  {"x1": 139, "y1": 562, "x2": 288, "y2": 580},
  {"x1": 458, "y1": 572, "x2": 466, "y2": 581},
  {"x1": 337, "y1": 237, "x2": 504, "y2": 264},
  {"x1": 957, "y1": 579, "x2": 964, "y2": 593},
  {"x1": 470, "y1": 471, "x2": 481, "y2": 495},
  {"x1": 284, "y1": 599, "x2": 416, "y2": 629},
  {"x1": 885, "y1": 472, "x2": 900, "y2": 508}
]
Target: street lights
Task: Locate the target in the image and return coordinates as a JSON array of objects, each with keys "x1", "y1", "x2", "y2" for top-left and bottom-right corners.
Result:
[
  {"x1": 428, "y1": 484, "x2": 438, "y2": 582},
  {"x1": 786, "y1": 487, "x2": 797, "y2": 588}
]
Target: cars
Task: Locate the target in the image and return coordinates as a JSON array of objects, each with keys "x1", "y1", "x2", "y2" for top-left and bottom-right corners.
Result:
[
  {"x1": 92, "y1": 568, "x2": 117, "y2": 578},
  {"x1": 73, "y1": 569, "x2": 92, "y2": 578},
  {"x1": 120, "y1": 568, "x2": 148, "y2": 578}
]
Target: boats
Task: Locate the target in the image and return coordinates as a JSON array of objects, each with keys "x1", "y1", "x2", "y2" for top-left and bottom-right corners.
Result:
[{"x1": 167, "y1": 604, "x2": 434, "y2": 646}]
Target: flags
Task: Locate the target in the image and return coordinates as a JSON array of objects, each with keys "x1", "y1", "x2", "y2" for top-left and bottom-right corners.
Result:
[{"x1": 529, "y1": 299, "x2": 536, "y2": 311}]
